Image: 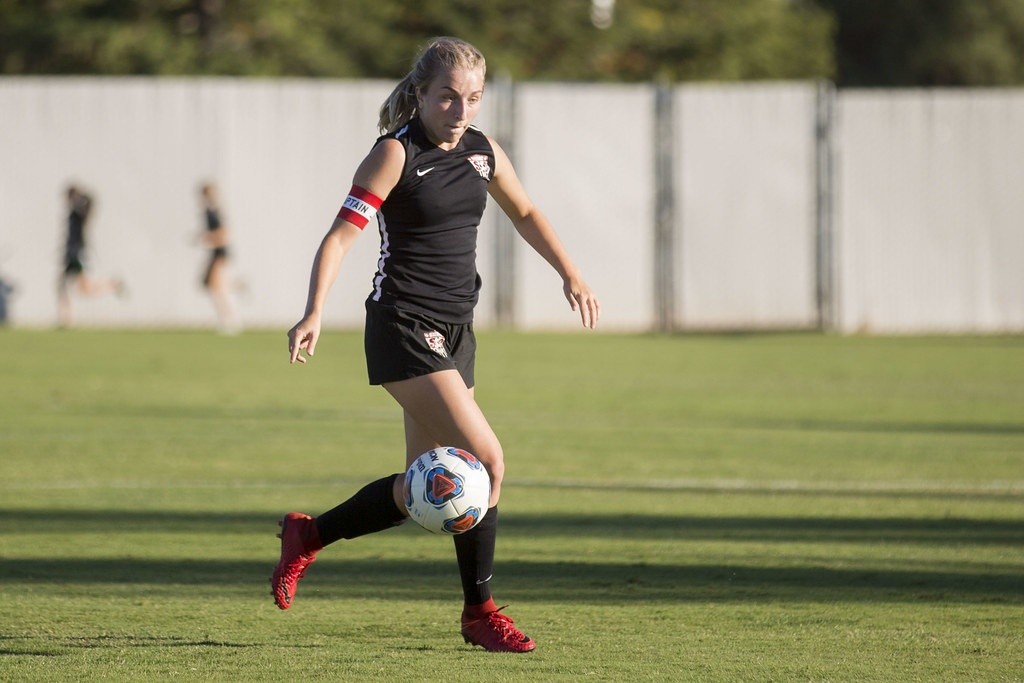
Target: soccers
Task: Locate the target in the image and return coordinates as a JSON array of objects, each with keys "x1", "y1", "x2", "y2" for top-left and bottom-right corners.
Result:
[{"x1": 405, "y1": 445, "x2": 492, "y2": 537}]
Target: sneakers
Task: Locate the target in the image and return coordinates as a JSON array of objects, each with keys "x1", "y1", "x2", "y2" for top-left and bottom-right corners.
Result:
[
  {"x1": 268, "y1": 513, "x2": 316, "y2": 610},
  {"x1": 461, "y1": 597, "x2": 536, "y2": 653}
]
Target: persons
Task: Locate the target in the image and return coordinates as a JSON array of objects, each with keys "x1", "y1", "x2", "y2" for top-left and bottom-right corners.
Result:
[
  {"x1": 197, "y1": 183, "x2": 239, "y2": 334},
  {"x1": 54, "y1": 186, "x2": 123, "y2": 328},
  {"x1": 271, "y1": 39, "x2": 601, "y2": 652}
]
